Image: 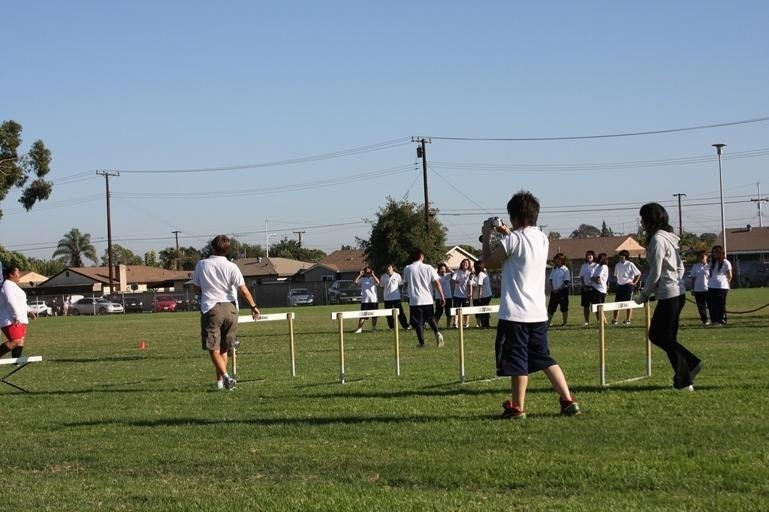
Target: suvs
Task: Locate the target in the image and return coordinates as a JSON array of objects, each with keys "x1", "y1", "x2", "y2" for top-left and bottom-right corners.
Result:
[{"x1": 487, "y1": 272, "x2": 501, "y2": 299}]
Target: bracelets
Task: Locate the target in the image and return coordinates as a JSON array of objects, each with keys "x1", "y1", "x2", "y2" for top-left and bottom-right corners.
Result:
[
  {"x1": 691, "y1": 289, "x2": 693, "y2": 291},
  {"x1": 250, "y1": 305, "x2": 257, "y2": 310}
]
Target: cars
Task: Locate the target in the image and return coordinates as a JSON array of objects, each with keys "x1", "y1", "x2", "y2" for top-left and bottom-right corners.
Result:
[
  {"x1": 328, "y1": 279, "x2": 362, "y2": 304},
  {"x1": 567, "y1": 259, "x2": 768, "y2": 295},
  {"x1": 26, "y1": 293, "x2": 200, "y2": 316},
  {"x1": 287, "y1": 288, "x2": 315, "y2": 308}
]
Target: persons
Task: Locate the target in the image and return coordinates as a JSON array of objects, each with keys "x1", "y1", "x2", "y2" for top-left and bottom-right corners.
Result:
[
  {"x1": 547, "y1": 253, "x2": 571, "y2": 327},
  {"x1": 690, "y1": 249, "x2": 711, "y2": 326},
  {"x1": 482, "y1": 190, "x2": 581, "y2": 419},
  {"x1": 50, "y1": 294, "x2": 71, "y2": 317},
  {"x1": 579, "y1": 251, "x2": 599, "y2": 326},
  {"x1": 613, "y1": 250, "x2": 642, "y2": 324},
  {"x1": 590, "y1": 253, "x2": 609, "y2": 323},
  {"x1": 707, "y1": 245, "x2": 732, "y2": 326},
  {"x1": 633, "y1": 202, "x2": 704, "y2": 392},
  {"x1": 192, "y1": 235, "x2": 259, "y2": 391},
  {"x1": 0, "y1": 265, "x2": 37, "y2": 368},
  {"x1": 353, "y1": 249, "x2": 492, "y2": 349}
]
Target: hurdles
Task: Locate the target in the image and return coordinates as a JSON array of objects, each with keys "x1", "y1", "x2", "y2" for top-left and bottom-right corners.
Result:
[
  {"x1": 331, "y1": 309, "x2": 401, "y2": 382},
  {"x1": 231, "y1": 313, "x2": 297, "y2": 378},
  {"x1": 449, "y1": 305, "x2": 500, "y2": 382},
  {"x1": 591, "y1": 301, "x2": 653, "y2": 386}
]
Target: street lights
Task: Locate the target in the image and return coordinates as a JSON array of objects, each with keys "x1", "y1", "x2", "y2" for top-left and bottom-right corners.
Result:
[
  {"x1": 172, "y1": 230, "x2": 183, "y2": 270},
  {"x1": 711, "y1": 143, "x2": 731, "y2": 257},
  {"x1": 265, "y1": 219, "x2": 278, "y2": 259},
  {"x1": 673, "y1": 193, "x2": 687, "y2": 235}
]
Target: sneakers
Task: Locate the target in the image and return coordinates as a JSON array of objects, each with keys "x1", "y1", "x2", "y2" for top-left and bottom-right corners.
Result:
[
  {"x1": 225, "y1": 378, "x2": 236, "y2": 390},
  {"x1": 207, "y1": 386, "x2": 223, "y2": 391},
  {"x1": 546, "y1": 319, "x2": 727, "y2": 328},
  {"x1": 560, "y1": 399, "x2": 581, "y2": 415},
  {"x1": 688, "y1": 362, "x2": 703, "y2": 379},
  {"x1": 12, "y1": 364, "x2": 20, "y2": 367},
  {"x1": 675, "y1": 385, "x2": 694, "y2": 393},
  {"x1": 500, "y1": 400, "x2": 527, "y2": 420},
  {"x1": 355, "y1": 321, "x2": 491, "y2": 348}
]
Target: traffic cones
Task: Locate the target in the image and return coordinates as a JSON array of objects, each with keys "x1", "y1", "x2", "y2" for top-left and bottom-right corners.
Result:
[{"x1": 138, "y1": 340, "x2": 146, "y2": 350}]
